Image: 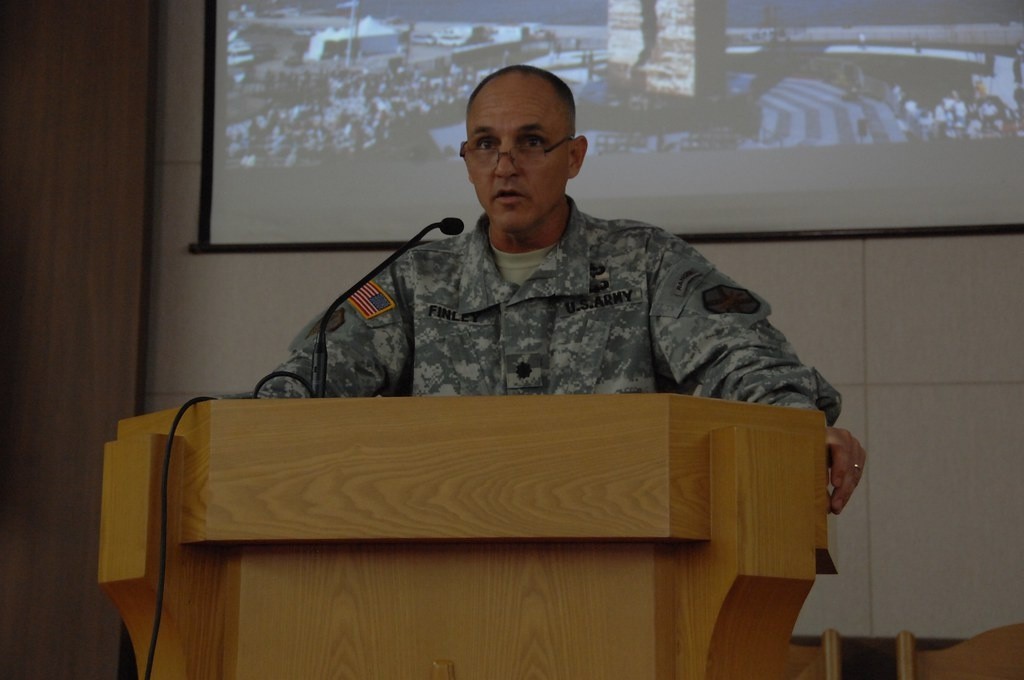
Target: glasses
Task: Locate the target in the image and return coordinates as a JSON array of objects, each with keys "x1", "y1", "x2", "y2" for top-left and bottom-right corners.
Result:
[{"x1": 460, "y1": 134, "x2": 574, "y2": 177}]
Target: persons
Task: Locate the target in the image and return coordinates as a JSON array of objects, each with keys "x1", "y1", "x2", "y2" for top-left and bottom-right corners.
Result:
[{"x1": 214, "y1": 66, "x2": 868, "y2": 516}]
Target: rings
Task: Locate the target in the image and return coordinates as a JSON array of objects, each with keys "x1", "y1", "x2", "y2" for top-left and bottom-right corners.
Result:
[{"x1": 851, "y1": 463, "x2": 862, "y2": 471}]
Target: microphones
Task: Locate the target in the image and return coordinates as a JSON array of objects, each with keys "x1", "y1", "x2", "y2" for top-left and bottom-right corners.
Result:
[{"x1": 316, "y1": 217, "x2": 465, "y2": 396}]
